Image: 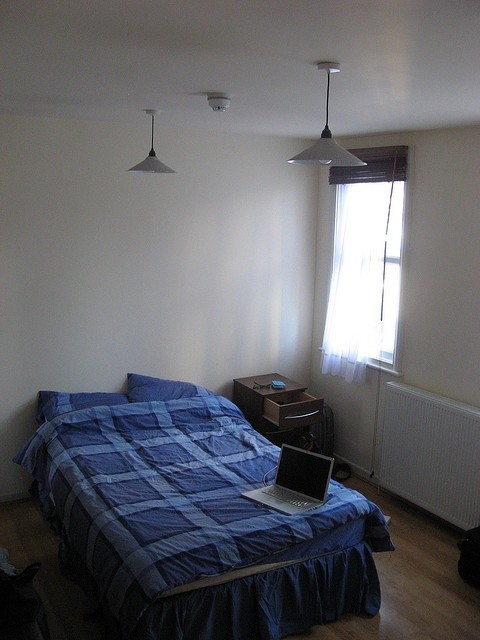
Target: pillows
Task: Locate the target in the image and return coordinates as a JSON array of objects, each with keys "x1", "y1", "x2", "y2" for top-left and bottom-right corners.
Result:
[
  {"x1": 126, "y1": 373, "x2": 212, "y2": 402},
  {"x1": 39, "y1": 390, "x2": 128, "y2": 422}
]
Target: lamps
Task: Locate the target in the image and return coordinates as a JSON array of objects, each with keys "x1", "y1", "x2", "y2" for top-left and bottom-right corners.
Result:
[
  {"x1": 209, "y1": 97, "x2": 231, "y2": 112},
  {"x1": 287, "y1": 62, "x2": 366, "y2": 167},
  {"x1": 128, "y1": 110, "x2": 177, "y2": 174}
]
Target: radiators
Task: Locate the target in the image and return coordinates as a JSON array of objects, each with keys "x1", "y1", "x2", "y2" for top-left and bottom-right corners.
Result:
[{"x1": 379, "y1": 381, "x2": 480, "y2": 531}]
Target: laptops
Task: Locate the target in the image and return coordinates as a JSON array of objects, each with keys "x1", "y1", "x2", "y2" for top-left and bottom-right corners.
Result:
[{"x1": 241, "y1": 443, "x2": 334, "y2": 516}]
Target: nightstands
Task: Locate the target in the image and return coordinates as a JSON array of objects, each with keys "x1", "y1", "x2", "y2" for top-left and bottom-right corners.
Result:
[{"x1": 234, "y1": 373, "x2": 324, "y2": 447}]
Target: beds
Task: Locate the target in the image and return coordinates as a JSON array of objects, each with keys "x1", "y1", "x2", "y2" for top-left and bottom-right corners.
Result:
[{"x1": 37, "y1": 392, "x2": 366, "y2": 635}]
